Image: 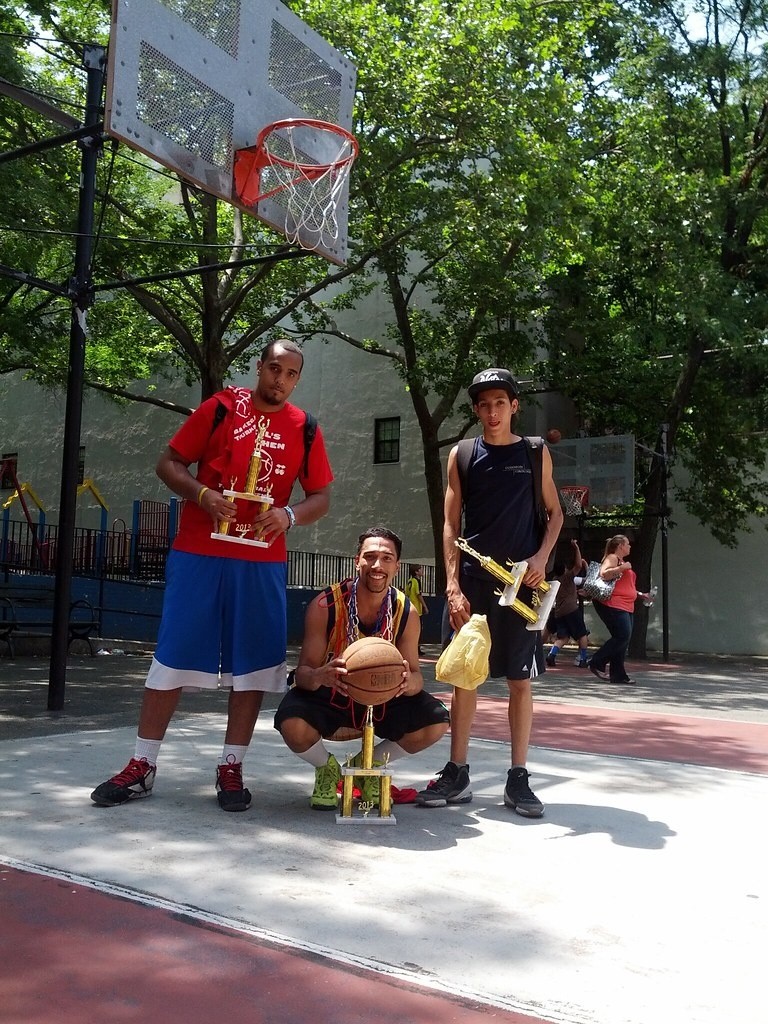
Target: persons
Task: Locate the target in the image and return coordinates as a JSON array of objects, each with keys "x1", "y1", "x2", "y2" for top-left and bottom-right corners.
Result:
[
  {"x1": 590, "y1": 534, "x2": 656, "y2": 684},
  {"x1": 415, "y1": 368, "x2": 564, "y2": 819},
  {"x1": 545, "y1": 538, "x2": 589, "y2": 668},
  {"x1": 405, "y1": 564, "x2": 428, "y2": 655},
  {"x1": 90, "y1": 339, "x2": 336, "y2": 813},
  {"x1": 273, "y1": 526, "x2": 450, "y2": 811}
]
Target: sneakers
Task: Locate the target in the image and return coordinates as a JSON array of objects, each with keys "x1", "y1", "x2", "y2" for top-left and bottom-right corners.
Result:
[
  {"x1": 503, "y1": 769, "x2": 546, "y2": 816},
  {"x1": 90, "y1": 752, "x2": 157, "y2": 806},
  {"x1": 215, "y1": 752, "x2": 251, "y2": 812},
  {"x1": 310, "y1": 752, "x2": 346, "y2": 807},
  {"x1": 414, "y1": 761, "x2": 473, "y2": 805},
  {"x1": 342, "y1": 750, "x2": 394, "y2": 809}
]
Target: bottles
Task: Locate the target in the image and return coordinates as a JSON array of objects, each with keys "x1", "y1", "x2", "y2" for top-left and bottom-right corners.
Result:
[{"x1": 643, "y1": 586, "x2": 658, "y2": 607}]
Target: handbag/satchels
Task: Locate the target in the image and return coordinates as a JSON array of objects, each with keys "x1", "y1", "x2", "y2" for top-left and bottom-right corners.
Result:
[{"x1": 583, "y1": 553, "x2": 623, "y2": 602}]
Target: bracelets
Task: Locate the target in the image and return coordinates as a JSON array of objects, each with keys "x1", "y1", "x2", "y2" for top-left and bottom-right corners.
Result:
[
  {"x1": 196, "y1": 485, "x2": 209, "y2": 505},
  {"x1": 284, "y1": 505, "x2": 296, "y2": 534}
]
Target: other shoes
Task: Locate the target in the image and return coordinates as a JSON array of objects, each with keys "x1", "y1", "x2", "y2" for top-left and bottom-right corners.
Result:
[
  {"x1": 610, "y1": 676, "x2": 636, "y2": 684},
  {"x1": 574, "y1": 655, "x2": 591, "y2": 667},
  {"x1": 419, "y1": 649, "x2": 424, "y2": 656},
  {"x1": 589, "y1": 663, "x2": 609, "y2": 680},
  {"x1": 547, "y1": 654, "x2": 558, "y2": 668}
]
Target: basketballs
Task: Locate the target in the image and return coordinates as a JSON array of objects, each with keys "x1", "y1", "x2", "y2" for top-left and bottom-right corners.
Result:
[{"x1": 340, "y1": 637, "x2": 405, "y2": 707}]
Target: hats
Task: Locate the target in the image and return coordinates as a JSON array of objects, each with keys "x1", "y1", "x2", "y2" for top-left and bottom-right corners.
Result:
[{"x1": 467, "y1": 368, "x2": 517, "y2": 402}]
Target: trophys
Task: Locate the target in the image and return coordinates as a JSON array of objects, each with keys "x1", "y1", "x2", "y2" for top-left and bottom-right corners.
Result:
[
  {"x1": 211, "y1": 415, "x2": 275, "y2": 549},
  {"x1": 455, "y1": 536, "x2": 561, "y2": 631}
]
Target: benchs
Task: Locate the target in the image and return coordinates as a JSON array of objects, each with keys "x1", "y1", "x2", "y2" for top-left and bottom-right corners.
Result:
[{"x1": 0, "y1": 583, "x2": 101, "y2": 660}]
